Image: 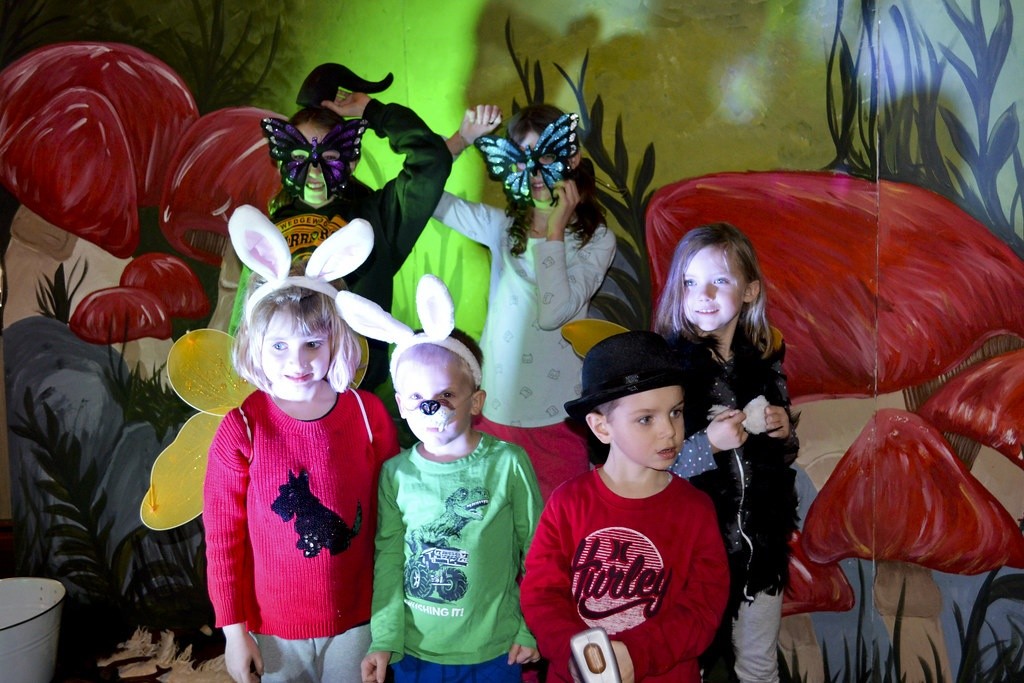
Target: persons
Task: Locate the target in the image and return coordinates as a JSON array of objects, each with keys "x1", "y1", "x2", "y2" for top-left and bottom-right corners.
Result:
[
  {"x1": 204, "y1": 256, "x2": 401, "y2": 683},
  {"x1": 263, "y1": 90, "x2": 454, "y2": 393},
  {"x1": 431, "y1": 103, "x2": 617, "y2": 506},
  {"x1": 655, "y1": 222, "x2": 801, "y2": 683},
  {"x1": 360, "y1": 327, "x2": 542, "y2": 683},
  {"x1": 519, "y1": 330, "x2": 730, "y2": 683}
]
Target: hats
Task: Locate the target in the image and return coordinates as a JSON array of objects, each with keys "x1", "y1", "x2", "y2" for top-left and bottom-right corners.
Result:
[{"x1": 563, "y1": 331, "x2": 696, "y2": 425}]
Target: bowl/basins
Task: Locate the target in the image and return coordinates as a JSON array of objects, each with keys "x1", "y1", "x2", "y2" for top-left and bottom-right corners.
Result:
[{"x1": 0, "y1": 575, "x2": 66, "y2": 683}]
